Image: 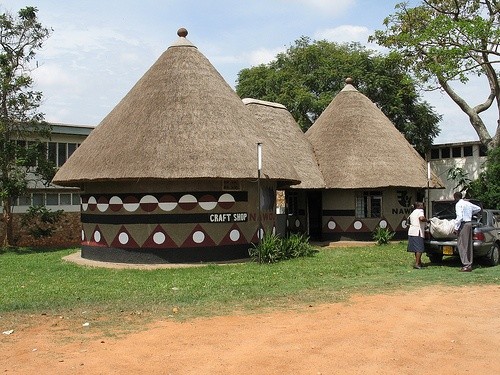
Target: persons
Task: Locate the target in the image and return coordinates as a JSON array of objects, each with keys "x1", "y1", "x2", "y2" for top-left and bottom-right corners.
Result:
[
  {"x1": 453, "y1": 192, "x2": 481, "y2": 272},
  {"x1": 406, "y1": 202, "x2": 430, "y2": 269}
]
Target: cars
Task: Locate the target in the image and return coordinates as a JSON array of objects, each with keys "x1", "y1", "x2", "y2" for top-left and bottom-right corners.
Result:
[{"x1": 424, "y1": 198, "x2": 500, "y2": 266}]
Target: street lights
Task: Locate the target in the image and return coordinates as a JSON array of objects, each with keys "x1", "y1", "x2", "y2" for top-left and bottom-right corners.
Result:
[
  {"x1": 256, "y1": 143, "x2": 263, "y2": 265},
  {"x1": 427, "y1": 161, "x2": 431, "y2": 219}
]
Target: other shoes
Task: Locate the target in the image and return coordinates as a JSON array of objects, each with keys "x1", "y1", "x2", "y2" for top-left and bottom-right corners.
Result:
[
  {"x1": 413, "y1": 264, "x2": 423, "y2": 269},
  {"x1": 459, "y1": 267, "x2": 471, "y2": 272}
]
